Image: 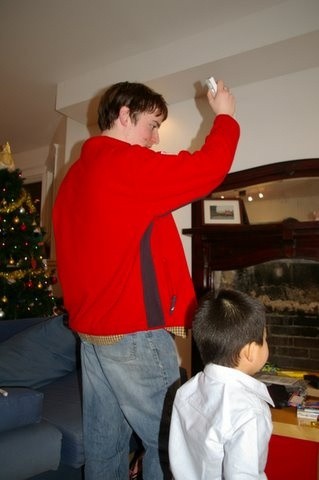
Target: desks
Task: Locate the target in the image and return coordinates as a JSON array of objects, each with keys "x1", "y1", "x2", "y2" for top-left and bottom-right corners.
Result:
[{"x1": 267, "y1": 369, "x2": 318, "y2": 480}]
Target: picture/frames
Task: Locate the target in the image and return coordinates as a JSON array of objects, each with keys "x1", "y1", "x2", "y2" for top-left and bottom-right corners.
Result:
[
  {"x1": 19, "y1": 171, "x2": 50, "y2": 234},
  {"x1": 202, "y1": 198, "x2": 243, "y2": 226}
]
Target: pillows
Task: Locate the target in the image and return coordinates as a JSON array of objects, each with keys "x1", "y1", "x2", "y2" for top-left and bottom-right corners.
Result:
[{"x1": 0, "y1": 311, "x2": 84, "y2": 389}]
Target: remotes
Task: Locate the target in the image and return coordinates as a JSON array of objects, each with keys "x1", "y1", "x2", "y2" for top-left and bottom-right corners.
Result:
[{"x1": 204, "y1": 75, "x2": 217, "y2": 97}]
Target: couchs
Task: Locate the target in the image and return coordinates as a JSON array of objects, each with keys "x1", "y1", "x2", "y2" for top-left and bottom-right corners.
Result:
[{"x1": 1, "y1": 316, "x2": 189, "y2": 480}]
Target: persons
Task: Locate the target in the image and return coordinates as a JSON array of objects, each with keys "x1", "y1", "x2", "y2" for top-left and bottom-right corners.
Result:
[
  {"x1": 166, "y1": 288, "x2": 276, "y2": 480},
  {"x1": 51, "y1": 79, "x2": 241, "y2": 480}
]
